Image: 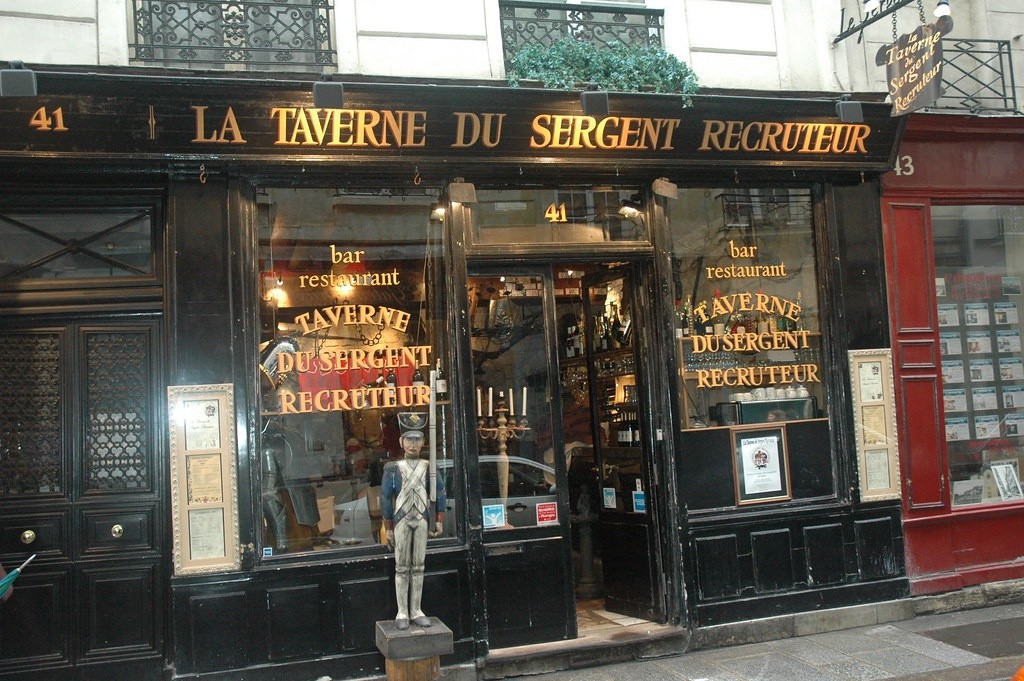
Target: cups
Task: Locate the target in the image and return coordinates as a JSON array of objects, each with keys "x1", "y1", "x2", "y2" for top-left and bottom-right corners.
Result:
[
  {"x1": 592, "y1": 353, "x2": 634, "y2": 377},
  {"x1": 729, "y1": 384, "x2": 808, "y2": 402}
]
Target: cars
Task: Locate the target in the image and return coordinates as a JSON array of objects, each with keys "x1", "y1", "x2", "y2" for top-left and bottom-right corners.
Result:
[{"x1": 387, "y1": 452, "x2": 569, "y2": 532}]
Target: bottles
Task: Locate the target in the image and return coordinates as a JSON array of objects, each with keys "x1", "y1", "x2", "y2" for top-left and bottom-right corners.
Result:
[
  {"x1": 682, "y1": 347, "x2": 823, "y2": 373},
  {"x1": 565, "y1": 301, "x2": 623, "y2": 359},
  {"x1": 616, "y1": 410, "x2": 640, "y2": 447},
  {"x1": 675, "y1": 288, "x2": 805, "y2": 338}
]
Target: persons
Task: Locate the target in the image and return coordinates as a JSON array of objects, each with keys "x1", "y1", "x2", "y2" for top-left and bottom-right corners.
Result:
[
  {"x1": 256, "y1": 376, "x2": 321, "y2": 554},
  {"x1": 379, "y1": 410, "x2": 448, "y2": 631}
]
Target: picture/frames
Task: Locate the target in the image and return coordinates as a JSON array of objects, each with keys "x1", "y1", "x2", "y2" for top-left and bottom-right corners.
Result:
[{"x1": 730, "y1": 422, "x2": 791, "y2": 506}]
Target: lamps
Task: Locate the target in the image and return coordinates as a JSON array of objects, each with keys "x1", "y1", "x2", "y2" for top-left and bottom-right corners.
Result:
[
  {"x1": 615, "y1": 193, "x2": 643, "y2": 219},
  {"x1": 580, "y1": 83, "x2": 609, "y2": 116},
  {"x1": 0, "y1": 60, "x2": 37, "y2": 97},
  {"x1": 652, "y1": 176, "x2": 678, "y2": 201},
  {"x1": 430, "y1": 195, "x2": 445, "y2": 220},
  {"x1": 447, "y1": 177, "x2": 477, "y2": 203},
  {"x1": 313, "y1": 73, "x2": 343, "y2": 108},
  {"x1": 835, "y1": 94, "x2": 864, "y2": 122}
]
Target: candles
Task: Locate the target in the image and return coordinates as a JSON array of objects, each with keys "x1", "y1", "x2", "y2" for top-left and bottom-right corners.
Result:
[
  {"x1": 509, "y1": 388, "x2": 514, "y2": 416},
  {"x1": 499, "y1": 391, "x2": 504, "y2": 397},
  {"x1": 522, "y1": 387, "x2": 527, "y2": 416},
  {"x1": 488, "y1": 386, "x2": 492, "y2": 417},
  {"x1": 477, "y1": 388, "x2": 482, "y2": 416}
]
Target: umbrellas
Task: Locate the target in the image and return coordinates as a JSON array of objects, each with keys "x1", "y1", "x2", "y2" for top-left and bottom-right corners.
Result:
[{"x1": 0, "y1": 554, "x2": 37, "y2": 599}]
